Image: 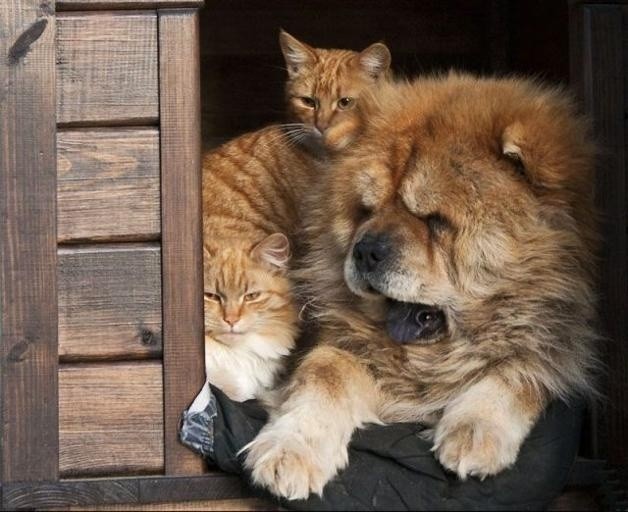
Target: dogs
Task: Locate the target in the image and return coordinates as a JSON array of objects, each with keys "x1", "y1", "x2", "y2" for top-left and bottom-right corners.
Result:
[{"x1": 235, "y1": 67, "x2": 613, "y2": 501}]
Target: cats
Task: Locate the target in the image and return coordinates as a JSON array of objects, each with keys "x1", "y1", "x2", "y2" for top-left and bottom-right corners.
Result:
[
  {"x1": 201, "y1": 121, "x2": 324, "y2": 402},
  {"x1": 256, "y1": 27, "x2": 391, "y2": 163}
]
